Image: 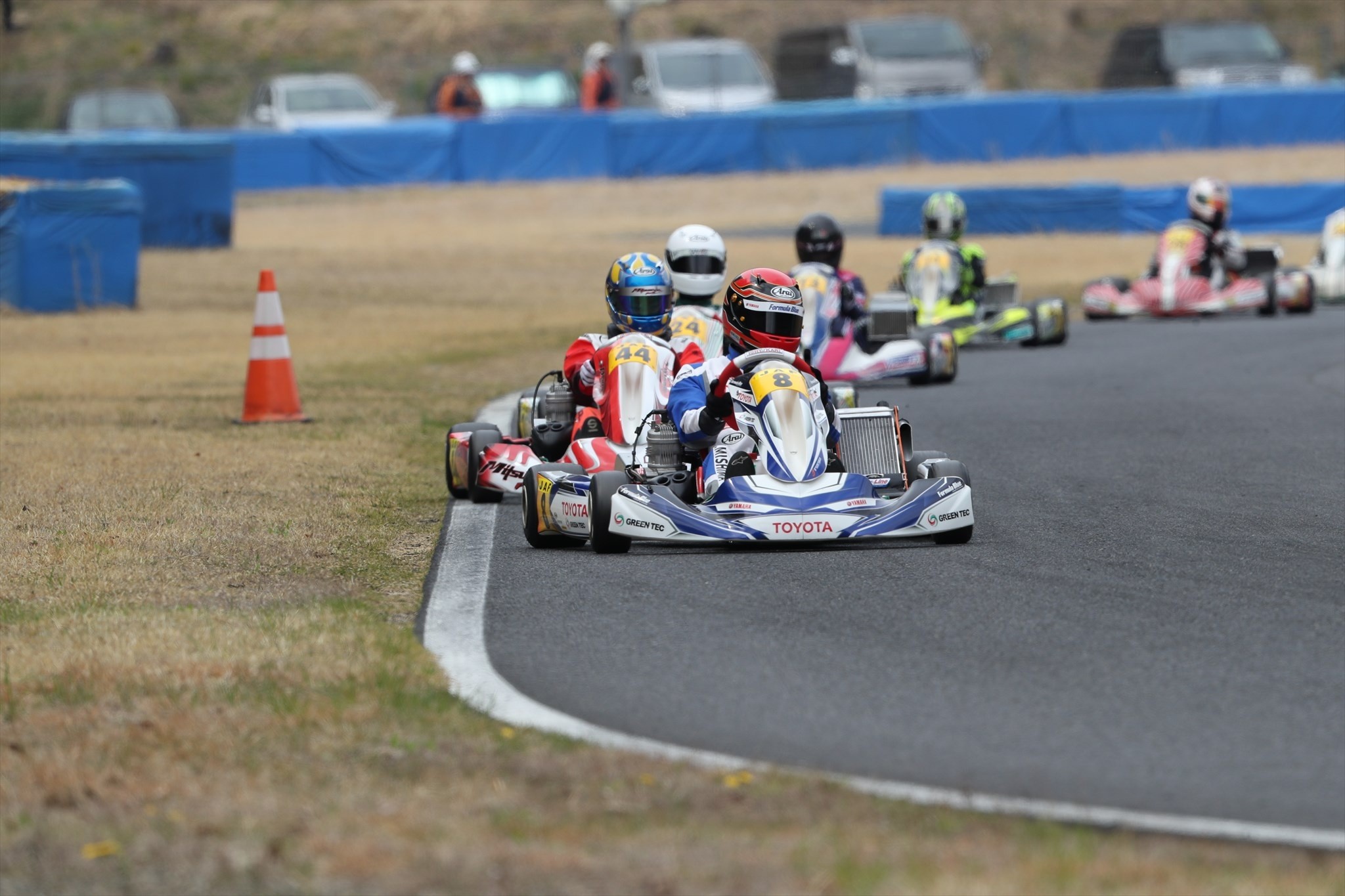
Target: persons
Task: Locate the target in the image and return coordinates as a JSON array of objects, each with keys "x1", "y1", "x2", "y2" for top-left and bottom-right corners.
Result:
[
  {"x1": 787, "y1": 214, "x2": 866, "y2": 354},
  {"x1": 1148, "y1": 176, "x2": 1247, "y2": 289},
  {"x1": 579, "y1": 41, "x2": 621, "y2": 109},
  {"x1": 666, "y1": 266, "x2": 847, "y2": 503},
  {"x1": 566, "y1": 251, "x2": 702, "y2": 446},
  {"x1": 434, "y1": 52, "x2": 483, "y2": 114},
  {"x1": 899, "y1": 193, "x2": 985, "y2": 308},
  {"x1": 664, "y1": 225, "x2": 726, "y2": 361}
]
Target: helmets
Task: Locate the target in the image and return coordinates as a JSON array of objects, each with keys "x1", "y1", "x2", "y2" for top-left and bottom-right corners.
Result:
[
  {"x1": 795, "y1": 213, "x2": 843, "y2": 267},
  {"x1": 923, "y1": 191, "x2": 967, "y2": 241},
  {"x1": 1188, "y1": 177, "x2": 1231, "y2": 223},
  {"x1": 664, "y1": 224, "x2": 727, "y2": 296},
  {"x1": 723, "y1": 267, "x2": 805, "y2": 354},
  {"x1": 604, "y1": 251, "x2": 675, "y2": 336}
]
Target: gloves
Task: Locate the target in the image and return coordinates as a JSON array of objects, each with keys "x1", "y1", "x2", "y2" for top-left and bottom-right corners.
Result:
[
  {"x1": 580, "y1": 359, "x2": 595, "y2": 386},
  {"x1": 810, "y1": 366, "x2": 829, "y2": 406},
  {"x1": 706, "y1": 379, "x2": 733, "y2": 418}
]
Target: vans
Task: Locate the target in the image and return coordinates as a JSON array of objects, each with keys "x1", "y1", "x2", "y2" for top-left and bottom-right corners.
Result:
[
  {"x1": 776, "y1": 14, "x2": 992, "y2": 101},
  {"x1": 610, "y1": 37, "x2": 783, "y2": 117}
]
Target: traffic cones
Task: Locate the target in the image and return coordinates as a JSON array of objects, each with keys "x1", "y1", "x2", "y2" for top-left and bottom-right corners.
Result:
[{"x1": 232, "y1": 269, "x2": 315, "y2": 426}]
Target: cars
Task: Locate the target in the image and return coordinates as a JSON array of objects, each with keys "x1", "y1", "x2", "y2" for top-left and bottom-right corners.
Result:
[
  {"x1": 433, "y1": 61, "x2": 582, "y2": 112},
  {"x1": 1095, "y1": 16, "x2": 1316, "y2": 88},
  {"x1": 237, "y1": 71, "x2": 402, "y2": 129},
  {"x1": 53, "y1": 84, "x2": 195, "y2": 139}
]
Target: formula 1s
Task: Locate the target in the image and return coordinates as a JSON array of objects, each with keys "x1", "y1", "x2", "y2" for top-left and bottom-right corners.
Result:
[
  {"x1": 1081, "y1": 219, "x2": 1316, "y2": 318},
  {"x1": 877, "y1": 238, "x2": 1070, "y2": 351},
  {"x1": 783, "y1": 261, "x2": 959, "y2": 385},
  {"x1": 445, "y1": 303, "x2": 975, "y2": 552}
]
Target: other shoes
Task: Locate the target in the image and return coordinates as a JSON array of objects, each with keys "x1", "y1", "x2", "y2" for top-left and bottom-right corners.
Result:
[
  {"x1": 724, "y1": 451, "x2": 756, "y2": 480},
  {"x1": 824, "y1": 451, "x2": 847, "y2": 472}
]
